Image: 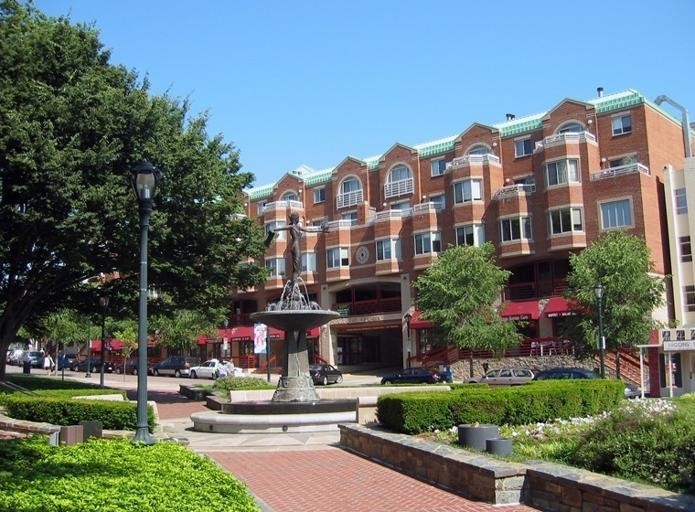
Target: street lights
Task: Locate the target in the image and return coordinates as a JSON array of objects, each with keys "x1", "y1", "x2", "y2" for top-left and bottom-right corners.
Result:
[
  {"x1": 593, "y1": 280, "x2": 607, "y2": 378},
  {"x1": 94, "y1": 285, "x2": 115, "y2": 387},
  {"x1": 220, "y1": 317, "x2": 230, "y2": 359},
  {"x1": 126, "y1": 149, "x2": 166, "y2": 449}
]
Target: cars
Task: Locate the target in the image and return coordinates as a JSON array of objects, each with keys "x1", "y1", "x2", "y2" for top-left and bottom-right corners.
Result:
[
  {"x1": 379, "y1": 366, "x2": 444, "y2": 386},
  {"x1": 532, "y1": 367, "x2": 602, "y2": 382},
  {"x1": 5, "y1": 346, "x2": 244, "y2": 381},
  {"x1": 309, "y1": 362, "x2": 343, "y2": 387}
]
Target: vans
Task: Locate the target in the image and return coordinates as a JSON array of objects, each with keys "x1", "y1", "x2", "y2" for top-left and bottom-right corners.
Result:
[{"x1": 463, "y1": 367, "x2": 536, "y2": 386}]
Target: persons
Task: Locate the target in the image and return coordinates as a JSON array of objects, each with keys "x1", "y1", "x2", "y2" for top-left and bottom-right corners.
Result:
[
  {"x1": 272, "y1": 211, "x2": 332, "y2": 300},
  {"x1": 441, "y1": 362, "x2": 456, "y2": 384},
  {"x1": 42, "y1": 350, "x2": 55, "y2": 377},
  {"x1": 216, "y1": 360, "x2": 223, "y2": 377},
  {"x1": 219, "y1": 361, "x2": 232, "y2": 376},
  {"x1": 482, "y1": 359, "x2": 489, "y2": 373},
  {"x1": 227, "y1": 358, "x2": 235, "y2": 376}
]
